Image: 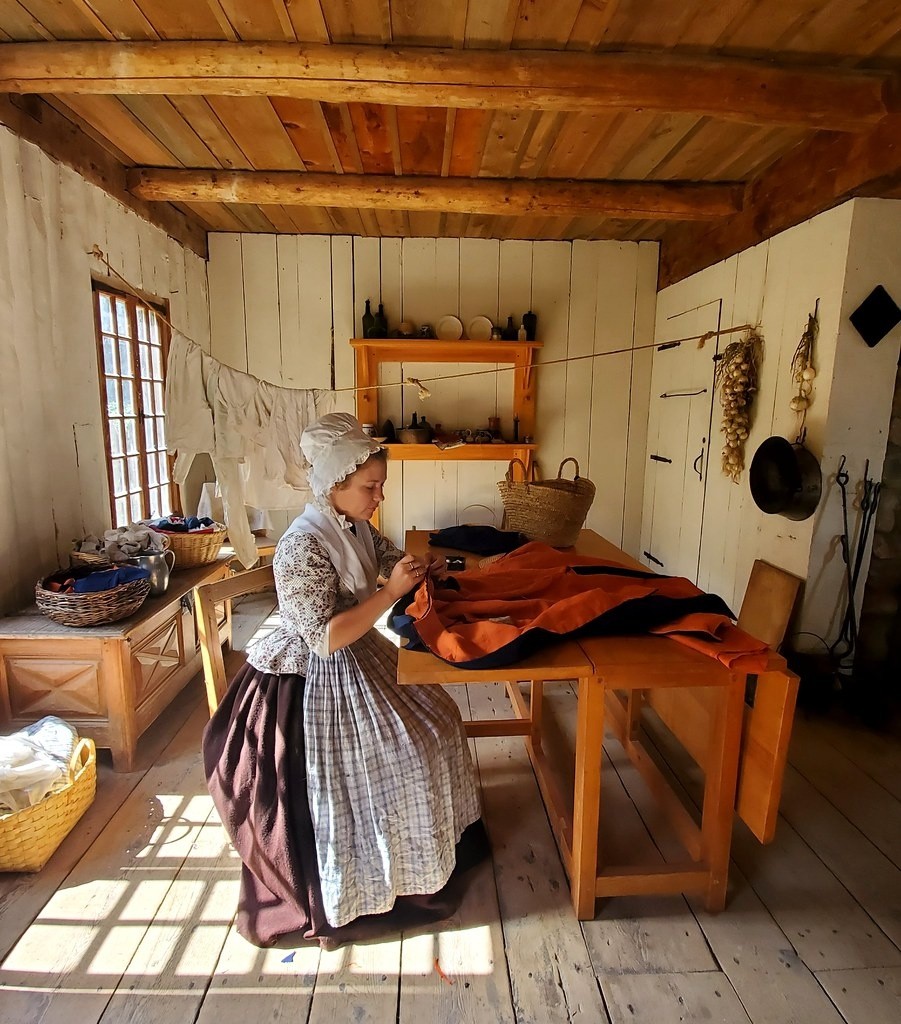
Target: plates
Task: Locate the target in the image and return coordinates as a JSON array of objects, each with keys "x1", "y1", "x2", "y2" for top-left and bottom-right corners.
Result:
[
  {"x1": 465, "y1": 316, "x2": 493, "y2": 340},
  {"x1": 371, "y1": 437, "x2": 388, "y2": 443},
  {"x1": 435, "y1": 316, "x2": 463, "y2": 340}
]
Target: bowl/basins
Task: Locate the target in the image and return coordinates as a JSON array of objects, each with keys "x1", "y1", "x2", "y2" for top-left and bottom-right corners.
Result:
[{"x1": 396, "y1": 427, "x2": 431, "y2": 444}]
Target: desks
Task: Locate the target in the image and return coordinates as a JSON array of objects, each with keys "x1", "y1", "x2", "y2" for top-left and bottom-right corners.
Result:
[{"x1": 394, "y1": 526, "x2": 799, "y2": 922}]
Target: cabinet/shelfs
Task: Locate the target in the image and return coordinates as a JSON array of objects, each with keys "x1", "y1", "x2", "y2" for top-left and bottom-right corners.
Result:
[
  {"x1": 0, "y1": 552, "x2": 237, "y2": 772},
  {"x1": 347, "y1": 337, "x2": 545, "y2": 534}
]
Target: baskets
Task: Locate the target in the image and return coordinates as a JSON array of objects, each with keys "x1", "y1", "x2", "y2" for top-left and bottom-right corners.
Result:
[
  {"x1": 35, "y1": 562, "x2": 150, "y2": 627},
  {"x1": 137, "y1": 519, "x2": 227, "y2": 569},
  {"x1": 0, "y1": 737, "x2": 97, "y2": 874},
  {"x1": 69, "y1": 532, "x2": 171, "y2": 566}
]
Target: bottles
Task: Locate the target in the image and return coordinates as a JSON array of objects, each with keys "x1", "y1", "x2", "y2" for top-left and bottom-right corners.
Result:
[
  {"x1": 375, "y1": 304, "x2": 387, "y2": 338},
  {"x1": 399, "y1": 323, "x2": 414, "y2": 337},
  {"x1": 418, "y1": 416, "x2": 431, "y2": 428},
  {"x1": 362, "y1": 300, "x2": 375, "y2": 338},
  {"x1": 407, "y1": 413, "x2": 420, "y2": 429},
  {"x1": 504, "y1": 311, "x2": 537, "y2": 342}
]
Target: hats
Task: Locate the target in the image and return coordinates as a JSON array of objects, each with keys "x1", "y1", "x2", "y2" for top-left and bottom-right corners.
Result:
[{"x1": 300, "y1": 413, "x2": 392, "y2": 500}]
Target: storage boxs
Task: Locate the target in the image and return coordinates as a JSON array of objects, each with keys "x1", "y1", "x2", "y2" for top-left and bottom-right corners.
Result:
[
  {"x1": 34, "y1": 562, "x2": 152, "y2": 627},
  {"x1": 140, "y1": 521, "x2": 227, "y2": 570},
  {"x1": 69, "y1": 543, "x2": 171, "y2": 567},
  {"x1": 0, "y1": 736, "x2": 97, "y2": 873}
]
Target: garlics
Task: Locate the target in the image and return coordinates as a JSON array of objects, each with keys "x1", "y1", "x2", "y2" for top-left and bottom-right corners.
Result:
[
  {"x1": 790, "y1": 355, "x2": 815, "y2": 411},
  {"x1": 722, "y1": 356, "x2": 757, "y2": 478}
]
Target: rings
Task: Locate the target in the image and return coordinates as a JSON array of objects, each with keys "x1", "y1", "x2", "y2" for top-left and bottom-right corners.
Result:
[
  {"x1": 409, "y1": 563, "x2": 414, "y2": 569},
  {"x1": 415, "y1": 569, "x2": 419, "y2": 577}
]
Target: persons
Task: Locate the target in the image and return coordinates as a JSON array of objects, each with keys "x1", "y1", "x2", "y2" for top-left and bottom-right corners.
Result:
[{"x1": 201, "y1": 412, "x2": 492, "y2": 951}]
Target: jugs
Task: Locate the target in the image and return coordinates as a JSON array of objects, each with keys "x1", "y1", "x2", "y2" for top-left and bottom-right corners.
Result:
[{"x1": 128, "y1": 549, "x2": 176, "y2": 595}]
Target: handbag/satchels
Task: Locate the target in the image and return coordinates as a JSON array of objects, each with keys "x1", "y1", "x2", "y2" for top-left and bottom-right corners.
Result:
[{"x1": 496, "y1": 456, "x2": 596, "y2": 549}]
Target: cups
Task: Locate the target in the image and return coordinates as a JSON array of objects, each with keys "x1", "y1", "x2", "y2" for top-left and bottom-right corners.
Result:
[
  {"x1": 489, "y1": 417, "x2": 499, "y2": 430},
  {"x1": 362, "y1": 423, "x2": 374, "y2": 436},
  {"x1": 490, "y1": 326, "x2": 502, "y2": 340}
]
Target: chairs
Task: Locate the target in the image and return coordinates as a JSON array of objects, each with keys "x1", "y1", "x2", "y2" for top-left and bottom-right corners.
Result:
[
  {"x1": 199, "y1": 482, "x2": 279, "y2": 569},
  {"x1": 193, "y1": 562, "x2": 279, "y2": 723}
]
